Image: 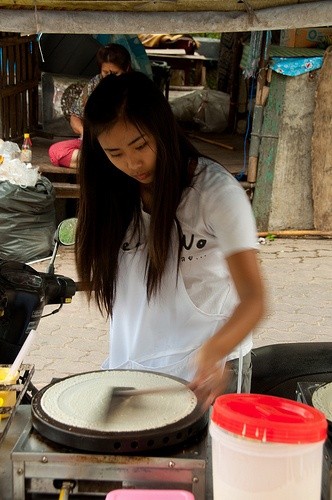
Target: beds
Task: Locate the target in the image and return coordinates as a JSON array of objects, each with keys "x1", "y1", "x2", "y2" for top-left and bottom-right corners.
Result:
[{"x1": 0, "y1": 34, "x2": 249, "y2": 228}]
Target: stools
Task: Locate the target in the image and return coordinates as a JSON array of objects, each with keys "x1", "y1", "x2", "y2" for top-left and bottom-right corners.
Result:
[{"x1": 151, "y1": 60, "x2": 172, "y2": 101}]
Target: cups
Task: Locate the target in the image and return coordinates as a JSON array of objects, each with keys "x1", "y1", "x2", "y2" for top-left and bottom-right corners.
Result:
[{"x1": 0, "y1": 363, "x2": 20, "y2": 419}]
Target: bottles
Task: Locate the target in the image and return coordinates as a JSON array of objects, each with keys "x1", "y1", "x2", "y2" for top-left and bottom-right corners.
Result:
[
  {"x1": 21, "y1": 134, "x2": 33, "y2": 163},
  {"x1": 208, "y1": 393, "x2": 327, "y2": 500}
]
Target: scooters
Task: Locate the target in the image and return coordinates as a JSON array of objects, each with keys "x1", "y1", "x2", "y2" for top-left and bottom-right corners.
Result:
[{"x1": 0, "y1": 217, "x2": 332, "y2": 450}]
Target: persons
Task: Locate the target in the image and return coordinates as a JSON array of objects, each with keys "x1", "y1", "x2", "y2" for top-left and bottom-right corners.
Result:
[
  {"x1": 75, "y1": 68, "x2": 266, "y2": 415},
  {"x1": 48, "y1": 33, "x2": 201, "y2": 170}
]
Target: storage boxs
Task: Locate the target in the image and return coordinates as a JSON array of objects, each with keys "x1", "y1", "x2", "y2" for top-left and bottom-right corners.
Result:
[
  {"x1": 280, "y1": 28, "x2": 332, "y2": 49},
  {"x1": 20, "y1": 72, "x2": 90, "y2": 137}
]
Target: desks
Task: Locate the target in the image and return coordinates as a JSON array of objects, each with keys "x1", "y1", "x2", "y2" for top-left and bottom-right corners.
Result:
[{"x1": 146, "y1": 53, "x2": 218, "y2": 87}]
